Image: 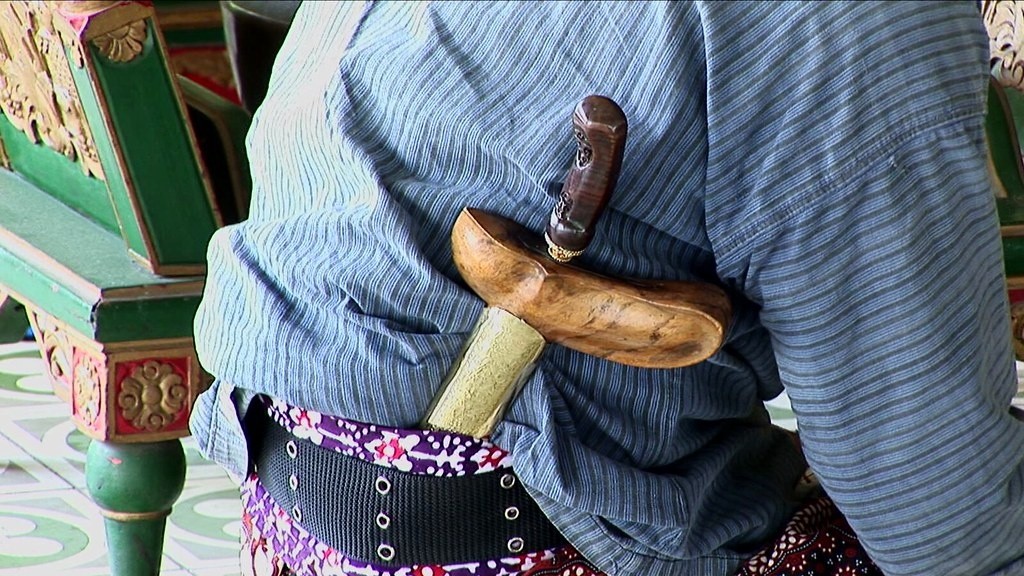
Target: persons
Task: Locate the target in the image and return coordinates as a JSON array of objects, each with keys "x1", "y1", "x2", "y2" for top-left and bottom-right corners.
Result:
[{"x1": 188, "y1": 0, "x2": 1024, "y2": 576}]
